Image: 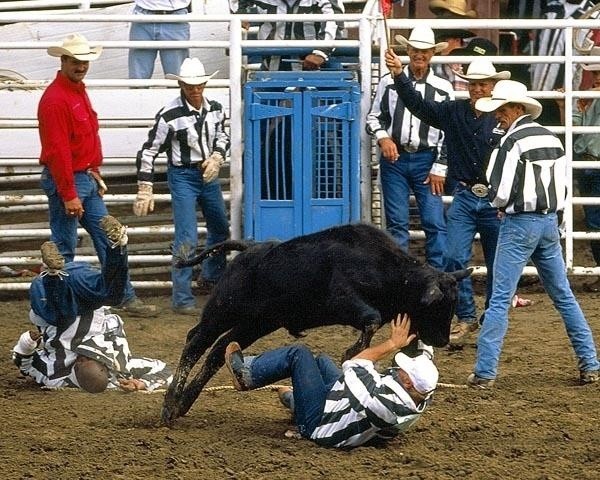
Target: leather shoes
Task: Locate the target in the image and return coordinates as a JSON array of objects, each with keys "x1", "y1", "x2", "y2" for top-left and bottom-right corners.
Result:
[
  {"x1": 116, "y1": 302, "x2": 163, "y2": 316},
  {"x1": 584, "y1": 277, "x2": 600, "y2": 291},
  {"x1": 451, "y1": 322, "x2": 477, "y2": 340},
  {"x1": 225, "y1": 341, "x2": 249, "y2": 392},
  {"x1": 278, "y1": 388, "x2": 294, "y2": 408},
  {"x1": 172, "y1": 306, "x2": 201, "y2": 316}
]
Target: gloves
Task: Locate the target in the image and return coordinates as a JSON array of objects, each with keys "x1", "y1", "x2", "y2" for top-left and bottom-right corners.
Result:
[
  {"x1": 133, "y1": 179, "x2": 154, "y2": 218},
  {"x1": 201, "y1": 152, "x2": 226, "y2": 184}
]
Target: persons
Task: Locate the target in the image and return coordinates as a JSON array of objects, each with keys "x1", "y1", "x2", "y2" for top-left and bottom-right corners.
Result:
[
  {"x1": 127, "y1": 0, "x2": 193, "y2": 129},
  {"x1": 227, "y1": 0, "x2": 348, "y2": 183},
  {"x1": 262, "y1": 82, "x2": 338, "y2": 200},
  {"x1": 9, "y1": 215, "x2": 175, "y2": 396},
  {"x1": 360, "y1": 0, "x2": 599, "y2": 294},
  {"x1": 131, "y1": 56, "x2": 232, "y2": 315},
  {"x1": 35, "y1": 30, "x2": 158, "y2": 321},
  {"x1": 383, "y1": 46, "x2": 513, "y2": 351},
  {"x1": 464, "y1": 78, "x2": 599, "y2": 392},
  {"x1": 223, "y1": 313, "x2": 440, "y2": 451}
]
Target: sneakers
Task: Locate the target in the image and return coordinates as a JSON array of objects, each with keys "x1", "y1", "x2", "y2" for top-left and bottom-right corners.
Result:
[
  {"x1": 100, "y1": 214, "x2": 125, "y2": 243},
  {"x1": 40, "y1": 240, "x2": 65, "y2": 271},
  {"x1": 580, "y1": 370, "x2": 599, "y2": 385},
  {"x1": 467, "y1": 373, "x2": 494, "y2": 389}
]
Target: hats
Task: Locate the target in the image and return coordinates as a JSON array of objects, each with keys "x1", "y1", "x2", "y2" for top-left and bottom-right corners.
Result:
[
  {"x1": 395, "y1": 351, "x2": 438, "y2": 395},
  {"x1": 577, "y1": 47, "x2": 600, "y2": 72},
  {"x1": 47, "y1": 33, "x2": 104, "y2": 62},
  {"x1": 164, "y1": 57, "x2": 219, "y2": 86},
  {"x1": 396, "y1": 0, "x2": 543, "y2": 120}
]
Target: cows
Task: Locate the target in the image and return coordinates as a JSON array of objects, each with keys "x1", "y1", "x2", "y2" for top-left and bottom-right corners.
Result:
[{"x1": 158, "y1": 221, "x2": 474, "y2": 427}]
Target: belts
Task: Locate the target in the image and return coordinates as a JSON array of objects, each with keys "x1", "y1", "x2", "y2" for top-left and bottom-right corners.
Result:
[
  {"x1": 396, "y1": 144, "x2": 433, "y2": 154},
  {"x1": 520, "y1": 210, "x2": 554, "y2": 217},
  {"x1": 182, "y1": 164, "x2": 203, "y2": 171},
  {"x1": 460, "y1": 181, "x2": 494, "y2": 199}
]
